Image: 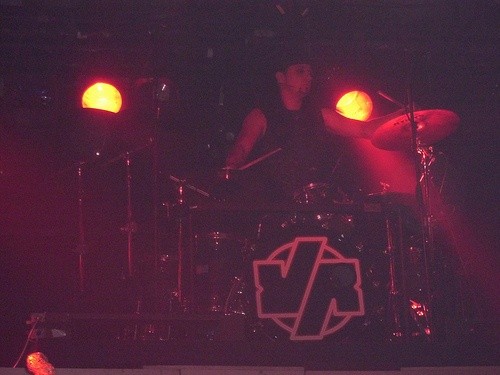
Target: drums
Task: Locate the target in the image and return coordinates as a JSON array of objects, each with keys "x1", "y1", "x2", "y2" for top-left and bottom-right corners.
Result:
[
  {"x1": 198, "y1": 228, "x2": 248, "y2": 311},
  {"x1": 295, "y1": 182, "x2": 336, "y2": 211},
  {"x1": 236, "y1": 216, "x2": 373, "y2": 353}
]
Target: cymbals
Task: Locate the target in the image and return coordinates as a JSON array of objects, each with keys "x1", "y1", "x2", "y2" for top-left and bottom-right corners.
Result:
[{"x1": 370, "y1": 109, "x2": 459, "y2": 150}]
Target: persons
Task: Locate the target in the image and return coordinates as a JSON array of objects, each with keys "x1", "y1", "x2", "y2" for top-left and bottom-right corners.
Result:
[{"x1": 224, "y1": 51, "x2": 418, "y2": 312}]
